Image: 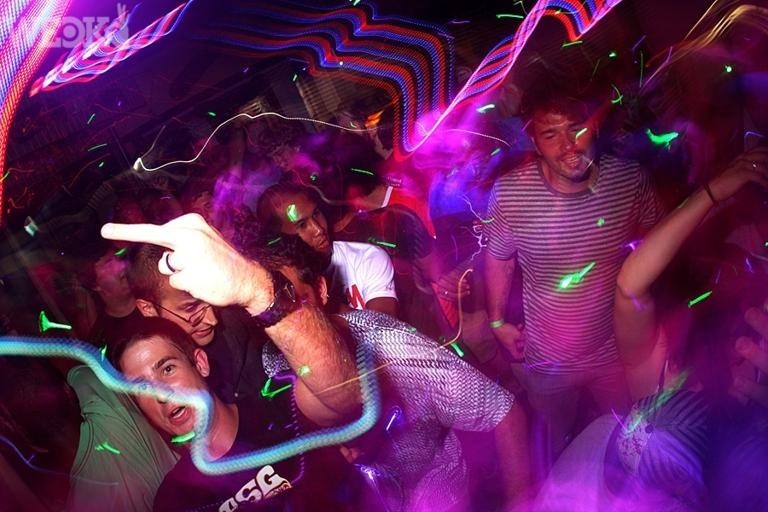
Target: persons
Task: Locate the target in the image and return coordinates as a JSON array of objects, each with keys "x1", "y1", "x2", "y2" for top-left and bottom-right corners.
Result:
[{"x1": 0, "y1": 27, "x2": 768, "y2": 512}]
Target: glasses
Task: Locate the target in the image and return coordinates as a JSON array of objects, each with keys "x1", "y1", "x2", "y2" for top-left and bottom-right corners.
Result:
[{"x1": 150, "y1": 299, "x2": 215, "y2": 327}]
